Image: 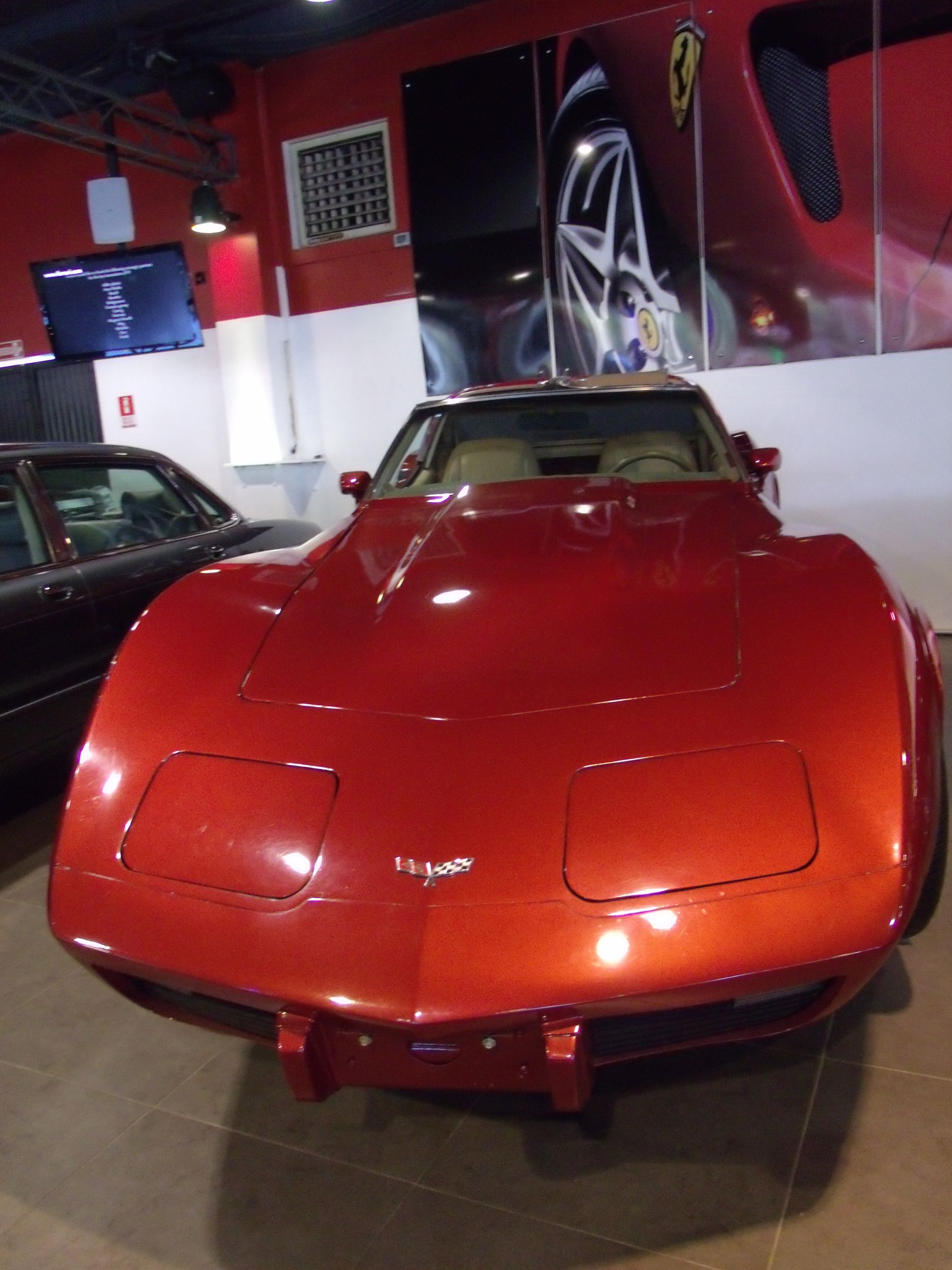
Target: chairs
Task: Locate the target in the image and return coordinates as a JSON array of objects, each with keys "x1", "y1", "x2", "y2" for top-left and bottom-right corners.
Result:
[
  {"x1": 442, "y1": 437, "x2": 542, "y2": 484},
  {"x1": 595, "y1": 430, "x2": 699, "y2": 475},
  {"x1": 0, "y1": 500, "x2": 35, "y2": 574}
]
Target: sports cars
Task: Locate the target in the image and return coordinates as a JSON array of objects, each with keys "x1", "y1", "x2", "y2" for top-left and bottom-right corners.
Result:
[{"x1": 43, "y1": 367, "x2": 951, "y2": 1116}]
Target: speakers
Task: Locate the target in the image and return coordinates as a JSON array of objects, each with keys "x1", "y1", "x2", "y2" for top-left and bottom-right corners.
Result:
[
  {"x1": 163, "y1": 67, "x2": 233, "y2": 121},
  {"x1": 86, "y1": 178, "x2": 135, "y2": 246}
]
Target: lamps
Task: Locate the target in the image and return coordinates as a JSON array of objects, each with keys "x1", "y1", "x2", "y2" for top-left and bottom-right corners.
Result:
[{"x1": 188, "y1": 183, "x2": 241, "y2": 235}]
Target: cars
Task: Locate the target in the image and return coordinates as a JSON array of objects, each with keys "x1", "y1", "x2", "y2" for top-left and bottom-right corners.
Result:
[{"x1": 0, "y1": 440, "x2": 325, "y2": 829}]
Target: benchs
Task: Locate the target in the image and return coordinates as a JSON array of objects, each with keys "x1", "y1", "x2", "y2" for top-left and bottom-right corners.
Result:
[{"x1": 63, "y1": 486, "x2": 197, "y2": 556}]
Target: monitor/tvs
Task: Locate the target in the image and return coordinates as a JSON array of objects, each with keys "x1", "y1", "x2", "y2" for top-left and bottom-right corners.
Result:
[{"x1": 28, "y1": 243, "x2": 203, "y2": 366}]
